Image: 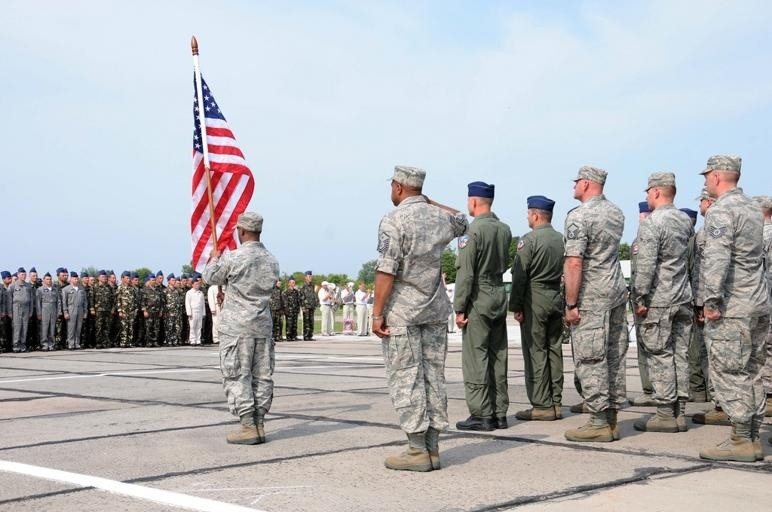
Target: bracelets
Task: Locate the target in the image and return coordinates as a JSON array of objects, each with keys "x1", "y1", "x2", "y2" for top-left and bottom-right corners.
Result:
[{"x1": 370, "y1": 313, "x2": 384, "y2": 320}]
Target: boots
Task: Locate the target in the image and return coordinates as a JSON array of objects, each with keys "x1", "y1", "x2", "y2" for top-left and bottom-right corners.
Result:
[
  {"x1": 384, "y1": 430, "x2": 440, "y2": 471},
  {"x1": 629, "y1": 392, "x2": 772, "y2": 463},
  {"x1": 457, "y1": 402, "x2": 619, "y2": 442},
  {"x1": 227, "y1": 415, "x2": 265, "y2": 445}
]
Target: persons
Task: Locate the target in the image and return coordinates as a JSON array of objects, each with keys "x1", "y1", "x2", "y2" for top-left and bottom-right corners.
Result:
[
  {"x1": 0, "y1": 265, "x2": 225, "y2": 353},
  {"x1": 445, "y1": 283, "x2": 458, "y2": 333},
  {"x1": 452, "y1": 180, "x2": 514, "y2": 433},
  {"x1": 201, "y1": 210, "x2": 280, "y2": 447},
  {"x1": 271, "y1": 271, "x2": 375, "y2": 338},
  {"x1": 508, "y1": 152, "x2": 772, "y2": 465},
  {"x1": 369, "y1": 164, "x2": 471, "y2": 472}
]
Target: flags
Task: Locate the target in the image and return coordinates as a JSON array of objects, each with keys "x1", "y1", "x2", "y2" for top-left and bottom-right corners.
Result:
[{"x1": 188, "y1": 63, "x2": 256, "y2": 277}]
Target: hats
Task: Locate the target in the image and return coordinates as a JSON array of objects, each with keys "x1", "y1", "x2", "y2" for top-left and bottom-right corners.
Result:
[
  {"x1": 304, "y1": 271, "x2": 312, "y2": 276},
  {"x1": 288, "y1": 276, "x2": 293, "y2": 280},
  {"x1": 571, "y1": 166, "x2": 607, "y2": 184},
  {"x1": 639, "y1": 156, "x2": 771, "y2": 216},
  {"x1": 467, "y1": 183, "x2": 494, "y2": 199},
  {"x1": 386, "y1": 164, "x2": 426, "y2": 188},
  {"x1": 231, "y1": 212, "x2": 262, "y2": 234},
  {"x1": 527, "y1": 195, "x2": 554, "y2": 211},
  {"x1": 2, "y1": 268, "x2": 202, "y2": 284}
]
